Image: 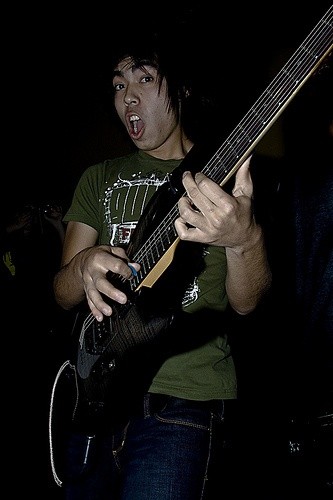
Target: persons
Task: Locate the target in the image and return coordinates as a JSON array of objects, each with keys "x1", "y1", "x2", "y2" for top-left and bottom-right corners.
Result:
[
  {"x1": 0, "y1": 195, "x2": 68, "y2": 276},
  {"x1": 51, "y1": 33, "x2": 273, "y2": 499}
]
